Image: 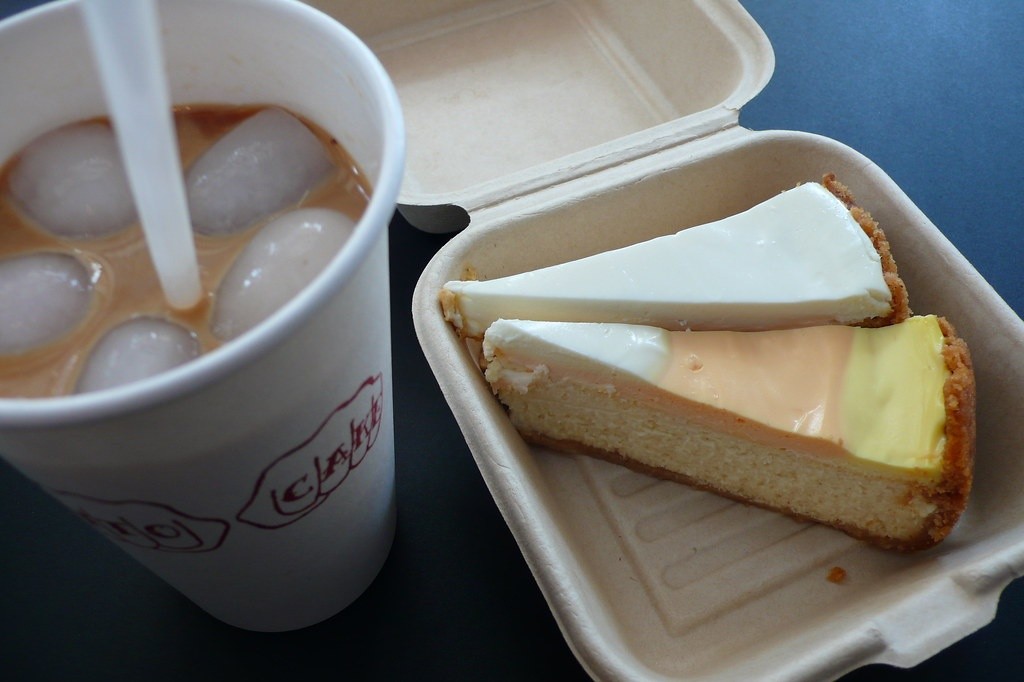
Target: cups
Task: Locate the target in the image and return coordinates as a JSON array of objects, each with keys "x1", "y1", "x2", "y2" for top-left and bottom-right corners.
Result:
[{"x1": 0, "y1": 0, "x2": 407, "y2": 635}]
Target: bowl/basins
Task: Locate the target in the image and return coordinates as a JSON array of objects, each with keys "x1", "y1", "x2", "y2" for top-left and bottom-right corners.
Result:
[{"x1": 298, "y1": 1, "x2": 1024, "y2": 682}]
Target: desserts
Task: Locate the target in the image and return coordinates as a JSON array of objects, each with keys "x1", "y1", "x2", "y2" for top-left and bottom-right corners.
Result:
[{"x1": 438, "y1": 179, "x2": 976, "y2": 553}]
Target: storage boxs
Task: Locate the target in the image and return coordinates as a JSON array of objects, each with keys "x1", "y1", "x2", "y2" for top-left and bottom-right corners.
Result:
[{"x1": 311, "y1": 0, "x2": 1024, "y2": 682}]
